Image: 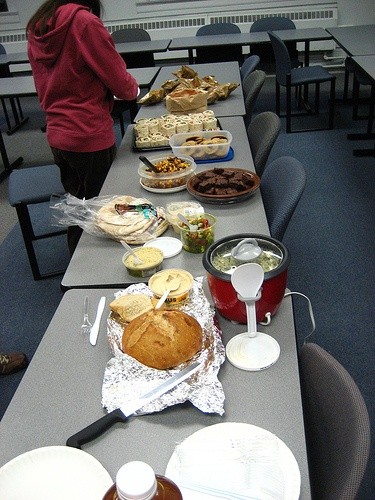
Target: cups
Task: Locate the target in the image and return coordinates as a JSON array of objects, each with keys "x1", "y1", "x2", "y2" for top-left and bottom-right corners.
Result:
[
  {"x1": 177, "y1": 213, "x2": 216, "y2": 253},
  {"x1": 147, "y1": 266, "x2": 194, "y2": 308}
]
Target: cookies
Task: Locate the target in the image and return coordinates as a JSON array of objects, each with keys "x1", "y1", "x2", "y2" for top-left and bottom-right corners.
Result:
[{"x1": 181, "y1": 135, "x2": 228, "y2": 157}]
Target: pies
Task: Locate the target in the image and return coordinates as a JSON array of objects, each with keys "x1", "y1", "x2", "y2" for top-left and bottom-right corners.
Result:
[{"x1": 96, "y1": 195, "x2": 169, "y2": 244}]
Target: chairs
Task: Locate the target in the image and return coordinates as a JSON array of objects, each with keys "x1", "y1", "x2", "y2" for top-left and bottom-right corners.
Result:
[
  {"x1": 249, "y1": 17, "x2": 300, "y2": 106},
  {"x1": 267, "y1": 31, "x2": 336, "y2": 134},
  {"x1": 240, "y1": 55, "x2": 260, "y2": 80},
  {"x1": 300, "y1": 343, "x2": 370, "y2": 500},
  {"x1": 261, "y1": 156, "x2": 306, "y2": 242},
  {"x1": 0, "y1": 44, "x2": 23, "y2": 120},
  {"x1": 195, "y1": 23, "x2": 245, "y2": 66},
  {"x1": 112, "y1": 28, "x2": 155, "y2": 68},
  {"x1": 247, "y1": 111, "x2": 281, "y2": 174},
  {"x1": 242, "y1": 70, "x2": 266, "y2": 122}
]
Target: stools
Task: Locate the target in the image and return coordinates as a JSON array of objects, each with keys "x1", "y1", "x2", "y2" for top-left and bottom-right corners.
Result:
[{"x1": 7, "y1": 164, "x2": 69, "y2": 281}]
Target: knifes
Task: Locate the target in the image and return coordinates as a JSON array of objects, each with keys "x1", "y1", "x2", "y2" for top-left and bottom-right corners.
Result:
[
  {"x1": 89, "y1": 296, "x2": 105, "y2": 345},
  {"x1": 65, "y1": 361, "x2": 201, "y2": 449}
]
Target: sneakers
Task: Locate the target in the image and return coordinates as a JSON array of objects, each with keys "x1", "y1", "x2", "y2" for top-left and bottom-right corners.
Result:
[{"x1": 0, "y1": 352, "x2": 28, "y2": 376}]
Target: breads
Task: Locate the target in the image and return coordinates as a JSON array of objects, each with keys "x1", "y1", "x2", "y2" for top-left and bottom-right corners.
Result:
[
  {"x1": 122, "y1": 308, "x2": 203, "y2": 369},
  {"x1": 109, "y1": 294, "x2": 153, "y2": 322}
]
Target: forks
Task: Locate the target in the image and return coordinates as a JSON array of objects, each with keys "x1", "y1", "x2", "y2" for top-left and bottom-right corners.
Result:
[
  {"x1": 120, "y1": 240, "x2": 143, "y2": 264},
  {"x1": 80, "y1": 296, "x2": 92, "y2": 334}
]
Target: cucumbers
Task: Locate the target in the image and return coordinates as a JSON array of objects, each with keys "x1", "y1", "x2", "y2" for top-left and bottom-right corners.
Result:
[{"x1": 182, "y1": 218, "x2": 214, "y2": 253}]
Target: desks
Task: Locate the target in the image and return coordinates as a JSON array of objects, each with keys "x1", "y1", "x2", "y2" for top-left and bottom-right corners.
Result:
[
  {"x1": 60, "y1": 116, "x2": 271, "y2": 294},
  {"x1": 167, "y1": 27, "x2": 333, "y2": 112},
  {"x1": 0, "y1": 289, "x2": 312, "y2": 500},
  {"x1": 133, "y1": 61, "x2": 246, "y2": 117},
  {"x1": 325, "y1": 26, "x2": 375, "y2": 156},
  {"x1": 0, "y1": 67, "x2": 161, "y2": 139},
  {"x1": 0, "y1": 39, "x2": 172, "y2": 70}
]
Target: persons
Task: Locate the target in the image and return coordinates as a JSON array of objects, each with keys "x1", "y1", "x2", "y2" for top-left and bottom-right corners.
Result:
[
  {"x1": 0, "y1": 352, "x2": 28, "y2": 375},
  {"x1": 26, "y1": 1, "x2": 140, "y2": 256}
]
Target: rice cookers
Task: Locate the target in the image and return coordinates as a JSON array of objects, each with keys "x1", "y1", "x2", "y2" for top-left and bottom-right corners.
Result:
[{"x1": 201, "y1": 233, "x2": 289, "y2": 323}]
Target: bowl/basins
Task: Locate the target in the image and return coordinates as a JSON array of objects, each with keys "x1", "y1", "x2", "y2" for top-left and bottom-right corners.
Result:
[
  {"x1": 168, "y1": 130, "x2": 232, "y2": 160},
  {"x1": 138, "y1": 154, "x2": 198, "y2": 190},
  {"x1": 121, "y1": 246, "x2": 163, "y2": 277},
  {"x1": 166, "y1": 200, "x2": 206, "y2": 234}
]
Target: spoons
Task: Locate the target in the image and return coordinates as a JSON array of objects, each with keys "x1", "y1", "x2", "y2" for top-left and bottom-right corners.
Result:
[
  {"x1": 154, "y1": 278, "x2": 180, "y2": 310},
  {"x1": 177, "y1": 213, "x2": 199, "y2": 230}
]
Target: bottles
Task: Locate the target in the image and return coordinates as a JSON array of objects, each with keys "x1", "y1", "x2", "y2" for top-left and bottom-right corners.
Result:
[{"x1": 101, "y1": 461, "x2": 182, "y2": 500}]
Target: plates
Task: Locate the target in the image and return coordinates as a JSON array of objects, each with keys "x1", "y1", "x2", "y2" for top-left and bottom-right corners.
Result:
[
  {"x1": 163, "y1": 422, "x2": 300, "y2": 500},
  {"x1": 185, "y1": 167, "x2": 261, "y2": 205},
  {"x1": 0, "y1": 446, "x2": 114, "y2": 500}
]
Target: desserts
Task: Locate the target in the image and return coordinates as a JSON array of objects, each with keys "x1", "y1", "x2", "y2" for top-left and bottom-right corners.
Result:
[
  {"x1": 191, "y1": 167, "x2": 253, "y2": 195},
  {"x1": 136, "y1": 110, "x2": 221, "y2": 148}
]
